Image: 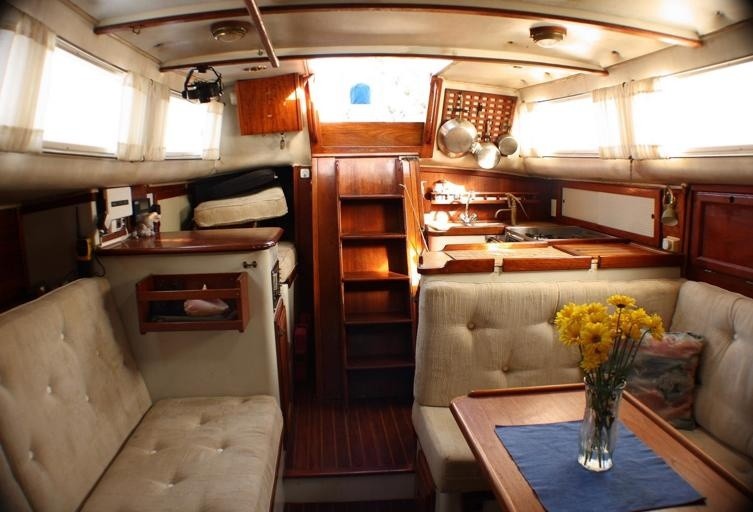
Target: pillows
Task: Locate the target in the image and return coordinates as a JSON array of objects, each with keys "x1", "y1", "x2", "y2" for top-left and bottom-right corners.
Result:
[{"x1": 605, "y1": 327, "x2": 706, "y2": 432}]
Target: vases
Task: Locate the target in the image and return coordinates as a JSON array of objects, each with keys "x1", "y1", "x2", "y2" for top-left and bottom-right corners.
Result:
[{"x1": 578, "y1": 373, "x2": 627, "y2": 472}]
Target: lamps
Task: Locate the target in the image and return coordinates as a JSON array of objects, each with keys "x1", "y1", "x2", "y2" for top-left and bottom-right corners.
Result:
[
  {"x1": 528, "y1": 25, "x2": 567, "y2": 48},
  {"x1": 211, "y1": 20, "x2": 252, "y2": 43}
]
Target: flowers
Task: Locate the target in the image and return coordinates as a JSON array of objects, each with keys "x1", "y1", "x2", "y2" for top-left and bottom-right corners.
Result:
[{"x1": 552, "y1": 294, "x2": 667, "y2": 468}]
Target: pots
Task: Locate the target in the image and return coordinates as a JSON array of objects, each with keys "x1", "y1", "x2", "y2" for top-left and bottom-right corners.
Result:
[{"x1": 438, "y1": 93, "x2": 519, "y2": 171}]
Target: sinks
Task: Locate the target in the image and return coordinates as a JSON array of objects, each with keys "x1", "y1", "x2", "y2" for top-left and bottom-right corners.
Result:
[{"x1": 444, "y1": 222, "x2": 501, "y2": 227}]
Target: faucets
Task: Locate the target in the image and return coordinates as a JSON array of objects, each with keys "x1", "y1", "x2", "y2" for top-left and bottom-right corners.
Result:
[{"x1": 494, "y1": 199, "x2": 518, "y2": 226}]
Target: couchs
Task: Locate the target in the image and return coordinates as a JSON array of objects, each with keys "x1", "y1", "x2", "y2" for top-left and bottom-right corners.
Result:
[
  {"x1": 409, "y1": 276, "x2": 752, "y2": 511},
  {"x1": 0, "y1": 274, "x2": 284, "y2": 511}
]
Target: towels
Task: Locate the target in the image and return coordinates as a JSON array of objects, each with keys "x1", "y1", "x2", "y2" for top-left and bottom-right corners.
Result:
[{"x1": 495, "y1": 419, "x2": 707, "y2": 511}]
